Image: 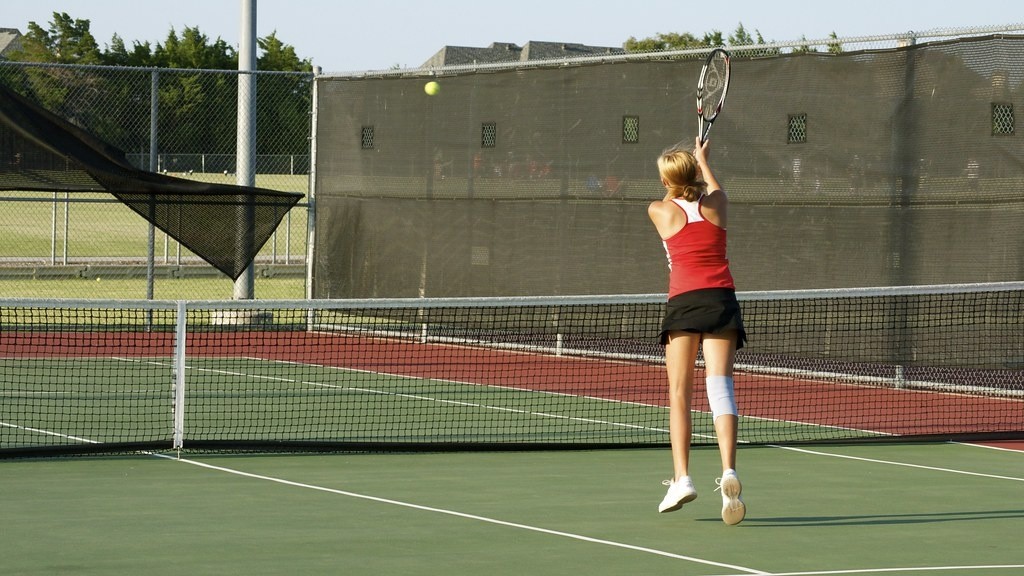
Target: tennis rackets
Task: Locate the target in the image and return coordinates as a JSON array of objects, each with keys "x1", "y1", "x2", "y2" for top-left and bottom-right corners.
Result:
[{"x1": 696, "y1": 49, "x2": 731, "y2": 146}]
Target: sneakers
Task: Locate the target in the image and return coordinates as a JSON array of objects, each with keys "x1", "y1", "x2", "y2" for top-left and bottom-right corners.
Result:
[
  {"x1": 659, "y1": 475, "x2": 697, "y2": 514},
  {"x1": 721, "y1": 469, "x2": 746, "y2": 525}
]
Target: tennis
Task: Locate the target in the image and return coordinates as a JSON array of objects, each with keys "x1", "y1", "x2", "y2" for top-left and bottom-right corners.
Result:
[{"x1": 424, "y1": 82, "x2": 440, "y2": 96}]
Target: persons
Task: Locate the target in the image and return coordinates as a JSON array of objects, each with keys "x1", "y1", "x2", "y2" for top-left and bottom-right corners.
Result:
[{"x1": 646, "y1": 134, "x2": 747, "y2": 524}]
